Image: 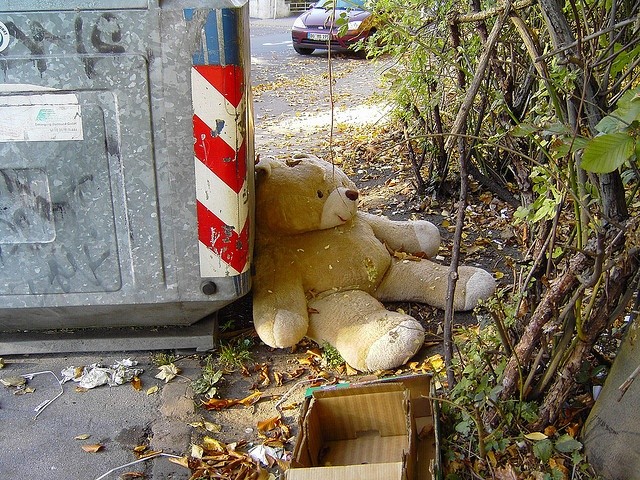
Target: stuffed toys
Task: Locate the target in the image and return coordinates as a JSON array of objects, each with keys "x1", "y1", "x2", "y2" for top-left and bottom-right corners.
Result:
[{"x1": 254, "y1": 155, "x2": 496, "y2": 372}]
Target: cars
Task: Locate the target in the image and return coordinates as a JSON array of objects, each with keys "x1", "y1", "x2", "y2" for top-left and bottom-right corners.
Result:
[{"x1": 291, "y1": 0, "x2": 402, "y2": 59}]
